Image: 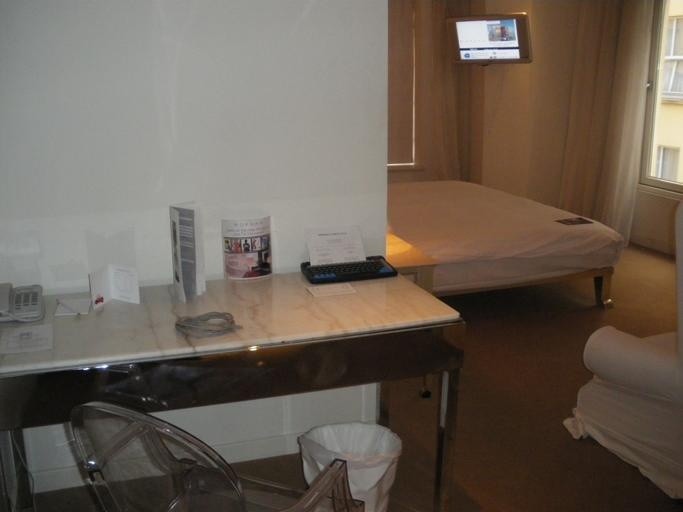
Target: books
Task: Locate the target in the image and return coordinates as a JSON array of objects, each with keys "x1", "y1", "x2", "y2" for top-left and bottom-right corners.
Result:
[
  {"x1": 555, "y1": 216, "x2": 595, "y2": 227},
  {"x1": 0, "y1": 321, "x2": 53, "y2": 355},
  {"x1": 167, "y1": 202, "x2": 207, "y2": 303},
  {"x1": 54, "y1": 298, "x2": 91, "y2": 316},
  {"x1": 87, "y1": 262, "x2": 141, "y2": 312}
]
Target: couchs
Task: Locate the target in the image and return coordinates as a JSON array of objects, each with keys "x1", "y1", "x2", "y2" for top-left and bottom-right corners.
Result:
[{"x1": 564, "y1": 198, "x2": 683, "y2": 501}]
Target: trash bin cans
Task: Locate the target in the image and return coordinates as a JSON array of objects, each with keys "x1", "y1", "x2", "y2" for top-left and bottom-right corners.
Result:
[{"x1": 298, "y1": 422, "x2": 402, "y2": 512}]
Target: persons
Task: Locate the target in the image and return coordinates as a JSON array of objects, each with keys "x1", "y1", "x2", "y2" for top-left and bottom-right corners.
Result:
[{"x1": 223, "y1": 238, "x2": 270, "y2": 254}]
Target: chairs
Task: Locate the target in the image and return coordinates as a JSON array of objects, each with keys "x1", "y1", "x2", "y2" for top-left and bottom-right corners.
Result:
[{"x1": 62, "y1": 401, "x2": 365, "y2": 512}]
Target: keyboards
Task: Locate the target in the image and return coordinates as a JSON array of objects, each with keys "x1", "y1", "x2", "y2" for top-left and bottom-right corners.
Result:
[{"x1": 301, "y1": 255, "x2": 397, "y2": 284}]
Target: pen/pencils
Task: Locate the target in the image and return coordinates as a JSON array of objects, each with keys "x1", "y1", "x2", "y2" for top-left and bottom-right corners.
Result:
[{"x1": 55, "y1": 298, "x2": 82, "y2": 317}]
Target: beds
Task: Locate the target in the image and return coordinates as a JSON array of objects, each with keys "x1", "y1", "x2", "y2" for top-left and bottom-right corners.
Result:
[{"x1": 387, "y1": 179, "x2": 626, "y2": 308}]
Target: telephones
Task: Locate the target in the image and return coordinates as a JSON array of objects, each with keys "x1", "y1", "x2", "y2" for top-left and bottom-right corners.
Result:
[{"x1": 0, "y1": 283, "x2": 45, "y2": 323}]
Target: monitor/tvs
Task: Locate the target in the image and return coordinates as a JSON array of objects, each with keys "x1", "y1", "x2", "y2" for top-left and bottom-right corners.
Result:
[{"x1": 445, "y1": 12, "x2": 532, "y2": 64}]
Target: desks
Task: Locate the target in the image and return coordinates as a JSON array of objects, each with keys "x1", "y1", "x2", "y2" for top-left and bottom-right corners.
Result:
[
  {"x1": 387, "y1": 234, "x2": 441, "y2": 398},
  {"x1": 0, "y1": 269, "x2": 464, "y2": 512}
]
